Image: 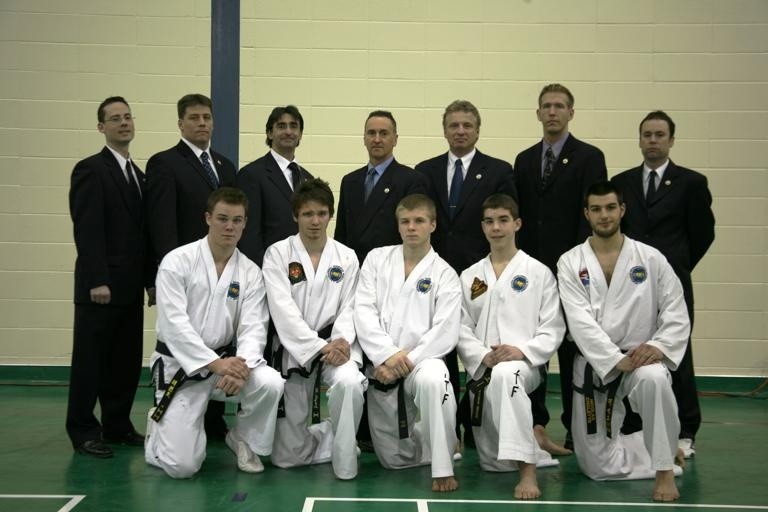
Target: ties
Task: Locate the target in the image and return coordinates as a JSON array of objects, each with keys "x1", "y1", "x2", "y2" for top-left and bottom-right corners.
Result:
[
  {"x1": 448, "y1": 159, "x2": 464, "y2": 222},
  {"x1": 287, "y1": 162, "x2": 301, "y2": 191},
  {"x1": 199, "y1": 151, "x2": 219, "y2": 191},
  {"x1": 542, "y1": 146, "x2": 556, "y2": 190},
  {"x1": 363, "y1": 168, "x2": 377, "y2": 204},
  {"x1": 646, "y1": 171, "x2": 657, "y2": 204},
  {"x1": 125, "y1": 160, "x2": 142, "y2": 208}
]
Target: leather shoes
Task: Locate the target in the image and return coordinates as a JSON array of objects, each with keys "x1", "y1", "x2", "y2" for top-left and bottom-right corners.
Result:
[
  {"x1": 563, "y1": 432, "x2": 574, "y2": 449},
  {"x1": 74, "y1": 436, "x2": 116, "y2": 457},
  {"x1": 100, "y1": 429, "x2": 150, "y2": 444}
]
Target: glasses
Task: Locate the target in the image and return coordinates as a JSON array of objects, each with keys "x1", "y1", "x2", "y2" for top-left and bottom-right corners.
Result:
[{"x1": 101, "y1": 115, "x2": 137, "y2": 124}]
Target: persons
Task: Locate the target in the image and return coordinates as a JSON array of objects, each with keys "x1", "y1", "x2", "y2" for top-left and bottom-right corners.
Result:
[
  {"x1": 556, "y1": 182, "x2": 696, "y2": 502},
  {"x1": 144, "y1": 94, "x2": 236, "y2": 441},
  {"x1": 334, "y1": 110, "x2": 433, "y2": 452},
  {"x1": 458, "y1": 193, "x2": 572, "y2": 500},
  {"x1": 262, "y1": 177, "x2": 369, "y2": 480},
  {"x1": 513, "y1": 83, "x2": 608, "y2": 450},
  {"x1": 415, "y1": 102, "x2": 518, "y2": 446},
  {"x1": 609, "y1": 110, "x2": 715, "y2": 459},
  {"x1": 144, "y1": 186, "x2": 284, "y2": 480},
  {"x1": 66, "y1": 94, "x2": 155, "y2": 458},
  {"x1": 235, "y1": 106, "x2": 315, "y2": 269},
  {"x1": 352, "y1": 193, "x2": 462, "y2": 492}
]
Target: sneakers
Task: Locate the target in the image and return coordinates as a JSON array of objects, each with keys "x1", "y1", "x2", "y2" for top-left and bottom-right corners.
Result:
[
  {"x1": 678, "y1": 437, "x2": 697, "y2": 461},
  {"x1": 224, "y1": 426, "x2": 266, "y2": 474},
  {"x1": 324, "y1": 416, "x2": 361, "y2": 458},
  {"x1": 144, "y1": 407, "x2": 157, "y2": 464}
]
